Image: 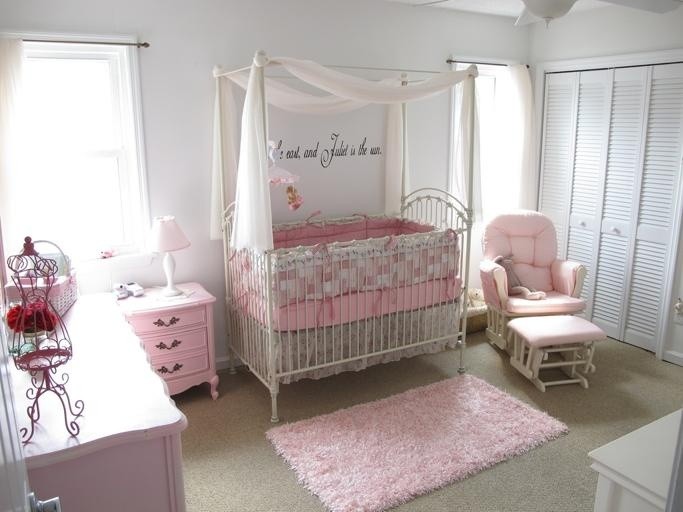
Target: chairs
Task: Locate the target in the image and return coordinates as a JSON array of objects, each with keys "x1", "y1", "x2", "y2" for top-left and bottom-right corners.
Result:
[{"x1": 479, "y1": 212, "x2": 586, "y2": 352}]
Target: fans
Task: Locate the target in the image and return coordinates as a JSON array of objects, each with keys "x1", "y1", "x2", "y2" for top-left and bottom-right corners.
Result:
[{"x1": 409, "y1": 0, "x2": 683, "y2": 29}]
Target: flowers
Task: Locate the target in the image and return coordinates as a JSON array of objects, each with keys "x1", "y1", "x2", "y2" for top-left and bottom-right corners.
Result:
[{"x1": 7, "y1": 302, "x2": 58, "y2": 335}]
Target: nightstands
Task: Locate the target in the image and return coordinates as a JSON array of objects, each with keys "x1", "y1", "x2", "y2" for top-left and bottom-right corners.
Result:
[{"x1": 115, "y1": 282, "x2": 221, "y2": 403}]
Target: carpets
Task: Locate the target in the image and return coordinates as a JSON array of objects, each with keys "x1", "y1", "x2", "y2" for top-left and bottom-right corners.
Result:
[{"x1": 264, "y1": 372, "x2": 570, "y2": 512}]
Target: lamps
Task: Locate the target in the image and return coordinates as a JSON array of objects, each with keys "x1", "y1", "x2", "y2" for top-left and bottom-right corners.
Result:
[
  {"x1": 523, "y1": 0, "x2": 578, "y2": 31},
  {"x1": 141, "y1": 216, "x2": 193, "y2": 299}
]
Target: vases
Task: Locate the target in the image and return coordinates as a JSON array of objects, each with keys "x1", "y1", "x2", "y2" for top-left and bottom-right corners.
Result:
[{"x1": 20, "y1": 330, "x2": 47, "y2": 346}]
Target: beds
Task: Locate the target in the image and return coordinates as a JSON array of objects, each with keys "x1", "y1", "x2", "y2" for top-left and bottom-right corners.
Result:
[{"x1": 210, "y1": 50, "x2": 479, "y2": 424}]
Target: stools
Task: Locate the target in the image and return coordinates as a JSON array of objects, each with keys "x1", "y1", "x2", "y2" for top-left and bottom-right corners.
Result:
[{"x1": 506, "y1": 314, "x2": 608, "y2": 393}]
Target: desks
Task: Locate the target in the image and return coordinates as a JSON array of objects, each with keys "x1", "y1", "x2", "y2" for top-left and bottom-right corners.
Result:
[
  {"x1": 8, "y1": 293, "x2": 189, "y2": 512},
  {"x1": 583, "y1": 409, "x2": 683, "y2": 512}
]
